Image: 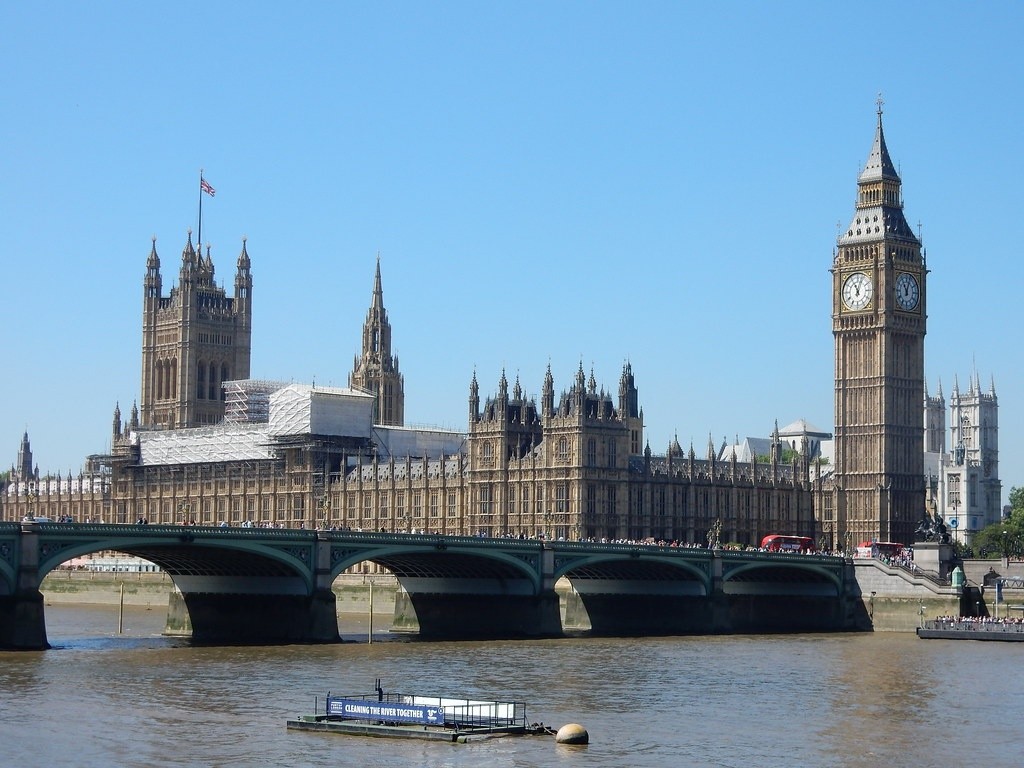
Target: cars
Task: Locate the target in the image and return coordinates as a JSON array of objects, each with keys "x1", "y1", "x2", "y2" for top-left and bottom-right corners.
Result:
[{"x1": 21, "y1": 517, "x2": 54, "y2": 532}]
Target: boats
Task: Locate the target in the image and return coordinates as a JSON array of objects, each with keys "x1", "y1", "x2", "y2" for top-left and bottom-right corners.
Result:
[
  {"x1": 916, "y1": 619, "x2": 1024, "y2": 642},
  {"x1": 147, "y1": 601, "x2": 152, "y2": 610},
  {"x1": 336, "y1": 609, "x2": 339, "y2": 618},
  {"x1": 45, "y1": 603, "x2": 52, "y2": 606}
]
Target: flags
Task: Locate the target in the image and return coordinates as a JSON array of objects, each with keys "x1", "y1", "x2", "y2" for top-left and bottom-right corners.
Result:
[{"x1": 200, "y1": 178, "x2": 215, "y2": 197}]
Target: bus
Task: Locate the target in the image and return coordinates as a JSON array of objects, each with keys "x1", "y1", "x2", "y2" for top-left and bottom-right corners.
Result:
[
  {"x1": 857, "y1": 542, "x2": 904, "y2": 557},
  {"x1": 761, "y1": 535, "x2": 817, "y2": 553}
]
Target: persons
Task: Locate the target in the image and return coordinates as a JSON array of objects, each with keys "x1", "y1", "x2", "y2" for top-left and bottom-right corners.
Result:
[
  {"x1": 378, "y1": 525, "x2": 487, "y2": 538},
  {"x1": 57, "y1": 513, "x2": 72, "y2": 522},
  {"x1": 496, "y1": 530, "x2": 628, "y2": 545},
  {"x1": 358, "y1": 525, "x2": 363, "y2": 532},
  {"x1": 853, "y1": 551, "x2": 917, "y2": 571},
  {"x1": 181, "y1": 519, "x2": 196, "y2": 527},
  {"x1": 745, "y1": 546, "x2": 845, "y2": 558},
  {"x1": 86, "y1": 515, "x2": 105, "y2": 524},
  {"x1": 297, "y1": 522, "x2": 352, "y2": 531},
  {"x1": 138, "y1": 516, "x2": 149, "y2": 525},
  {"x1": 220, "y1": 519, "x2": 286, "y2": 529},
  {"x1": 934, "y1": 614, "x2": 1024, "y2": 632},
  {"x1": 628, "y1": 534, "x2": 741, "y2": 551}
]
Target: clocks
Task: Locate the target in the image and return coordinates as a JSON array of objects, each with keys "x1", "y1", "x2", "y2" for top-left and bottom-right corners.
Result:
[
  {"x1": 842, "y1": 273, "x2": 873, "y2": 310},
  {"x1": 895, "y1": 272, "x2": 920, "y2": 311}
]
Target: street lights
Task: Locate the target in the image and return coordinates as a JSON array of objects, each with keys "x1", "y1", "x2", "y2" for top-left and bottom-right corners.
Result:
[
  {"x1": 544, "y1": 508, "x2": 555, "y2": 541},
  {"x1": 844, "y1": 528, "x2": 852, "y2": 558},
  {"x1": 870, "y1": 592, "x2": 876, "y2": 631},
  {"x1": 706, "y1": 529, "x2": 714, "y2": 550},
  {"x1": 402, "y1": 512, "x2": 413, "y2": 534},
  {"x1": 319, "y1": 494, "x2": 331, "y2": 531},
  {"x1": 177, "y1": 498, "x2": 190, "y2": 526},
  {"x1": 714, "y1": 518, "x2": 723, "y2": 549},
  {"x1": 22, "y1": 482, "x2": 39, "y2": 521},
  {"x1": 818, "y1": 538, "x2": 826, "y2": 551},
  {"x1": 369, "y1": 579, "x2": 375, "y2": 644},
  {"x1": 1002, "y1": 529, "x2": 1008, "y2": 558},
  {"x1": 952, "y1": 498, "x2": 962, "y2": 562},
  {"x1": 1017, "y1": 534, "x2": 1021, "y2": 561}
]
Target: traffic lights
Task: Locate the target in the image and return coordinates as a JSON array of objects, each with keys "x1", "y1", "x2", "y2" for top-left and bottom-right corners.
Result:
[
  {"x1": 969, "y1": 548, "x2": 972, "y2": 554},
  {"x1": 983, "y1": 549, "x2": 986, "y2": 555}
]
